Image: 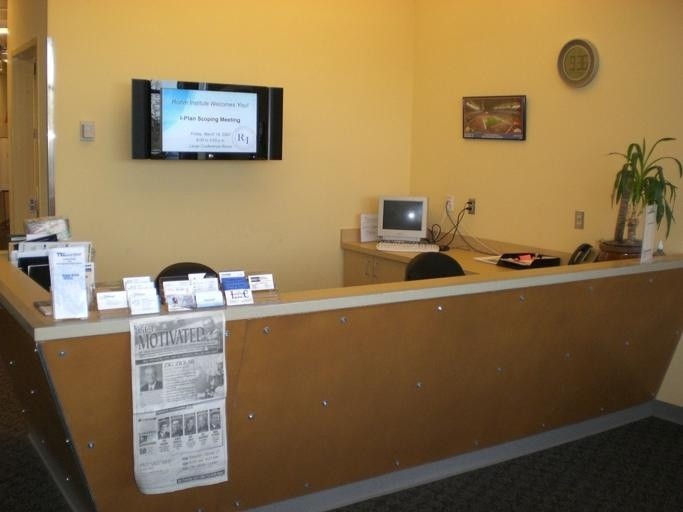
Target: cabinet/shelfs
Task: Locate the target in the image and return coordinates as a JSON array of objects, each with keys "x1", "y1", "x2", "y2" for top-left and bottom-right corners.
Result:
[{"x1": 343, "y1": 250, "x2": 404, "y2": 287}]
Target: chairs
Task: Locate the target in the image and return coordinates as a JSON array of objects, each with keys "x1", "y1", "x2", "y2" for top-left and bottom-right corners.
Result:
[
  {"x1": 404, "y1": 251, "x2": 464, "y2": 282},
  {"x1": 153, "y1": 262, "x2": 219, "y2": 296}
]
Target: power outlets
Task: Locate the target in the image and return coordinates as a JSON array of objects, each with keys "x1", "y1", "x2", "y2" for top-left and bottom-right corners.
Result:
[{"x1": 466, "y1": 198, "x2": 475, "y2": 215}]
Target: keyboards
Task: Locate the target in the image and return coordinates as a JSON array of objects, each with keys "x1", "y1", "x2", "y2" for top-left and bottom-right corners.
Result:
[{"x1": 375, "y1": 241, "x2": 440, "y2": 253}]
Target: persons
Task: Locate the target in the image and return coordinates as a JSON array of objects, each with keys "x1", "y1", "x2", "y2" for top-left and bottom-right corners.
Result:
[
  {"x1": 170, "y1": 420, "x2": 183, "y2": 437},
  {"x1": 209, "y1": 413, "x2": 221, "y2": 431},
  {"x1": 197, "y1": 414, "x2": 207, "y2": 434},
  {"x1": 157, "y1": 420, "x2": 169, "y2": 440},
  {"x1": 184, "y1": 417, "x2": 196, "y2": 436},
  {"x1": 139, "y1": 365, "x2": 162, "y2": 390}
]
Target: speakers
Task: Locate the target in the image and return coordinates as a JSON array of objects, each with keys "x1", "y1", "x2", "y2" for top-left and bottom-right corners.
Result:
[
  {"x1": 268, "y1": 86, "x2": 284, "y2": 161},
  {"x1": 131, "y1": 77, "x2": 148, "y2": 159}
]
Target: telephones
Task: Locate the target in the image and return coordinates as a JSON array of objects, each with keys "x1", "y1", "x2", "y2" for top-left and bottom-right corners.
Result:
[{"x1": 567, "y1": 243, "x2": 600, "y2": 264}]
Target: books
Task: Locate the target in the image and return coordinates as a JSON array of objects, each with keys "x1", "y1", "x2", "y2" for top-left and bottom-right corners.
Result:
[
  {"x1": 360, "y1": 212, "x2": 379, "y2": 244},
  {"x1": 585, "y1": 246, "x2": 602, "y2": 263},
  {"x1": 7, "y1": 213, "x2": 253, "y2": 318},
  {"x1": 472, "y1": 255, "x2": 502, "y2": 265}
]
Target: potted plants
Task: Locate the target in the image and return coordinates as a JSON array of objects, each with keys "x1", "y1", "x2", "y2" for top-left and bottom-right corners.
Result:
[{"x1": 598, "y1": 136, "x2": 683, "y2": 260}]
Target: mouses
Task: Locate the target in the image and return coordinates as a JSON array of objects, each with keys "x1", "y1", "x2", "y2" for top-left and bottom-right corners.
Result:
[{"x1": 440, "y1": 245, "x2": 449, "y2": 251}]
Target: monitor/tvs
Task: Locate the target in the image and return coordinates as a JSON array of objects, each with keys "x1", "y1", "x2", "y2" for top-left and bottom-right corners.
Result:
[
  {"x1": 377, "y1": 195, "x2": 428, "y2": 242},
  {"x1": 148, "y1": 80, "x2": 269, "y2": 161}
]
